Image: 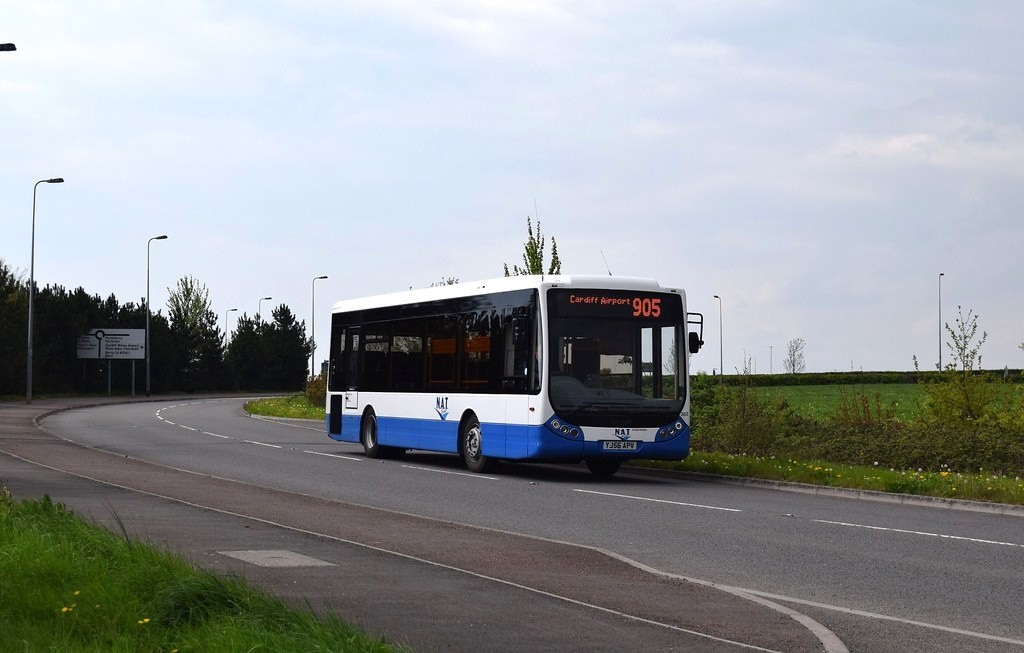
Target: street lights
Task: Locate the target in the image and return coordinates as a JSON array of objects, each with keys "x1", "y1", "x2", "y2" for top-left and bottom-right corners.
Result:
[
  {"x1": 26, "y1": 177, "x2": 64, "y2": 405},
  {"x1": 258, "y1": 297, "x2": 272, "y2": 323},
  {"x1": 938, "y1": 272, "x2": 944, "y2": 376},
  {"x1": 713, "y1": 295, "x2": 723, "y2": 381},
  {"x1": 145, "y1": 235, "x2": 168, "y2": 396},
  {"x1": 224, "y1": 308, "x2": 237, "y2": 348},
  {"x1": 310, "y1": 276, "x2": 329, "y2": 381}
]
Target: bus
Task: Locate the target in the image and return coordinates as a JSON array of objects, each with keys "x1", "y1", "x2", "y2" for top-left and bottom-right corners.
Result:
[{"x1": 325, "y1": 275, "x2": 706, "y2": 474}]
